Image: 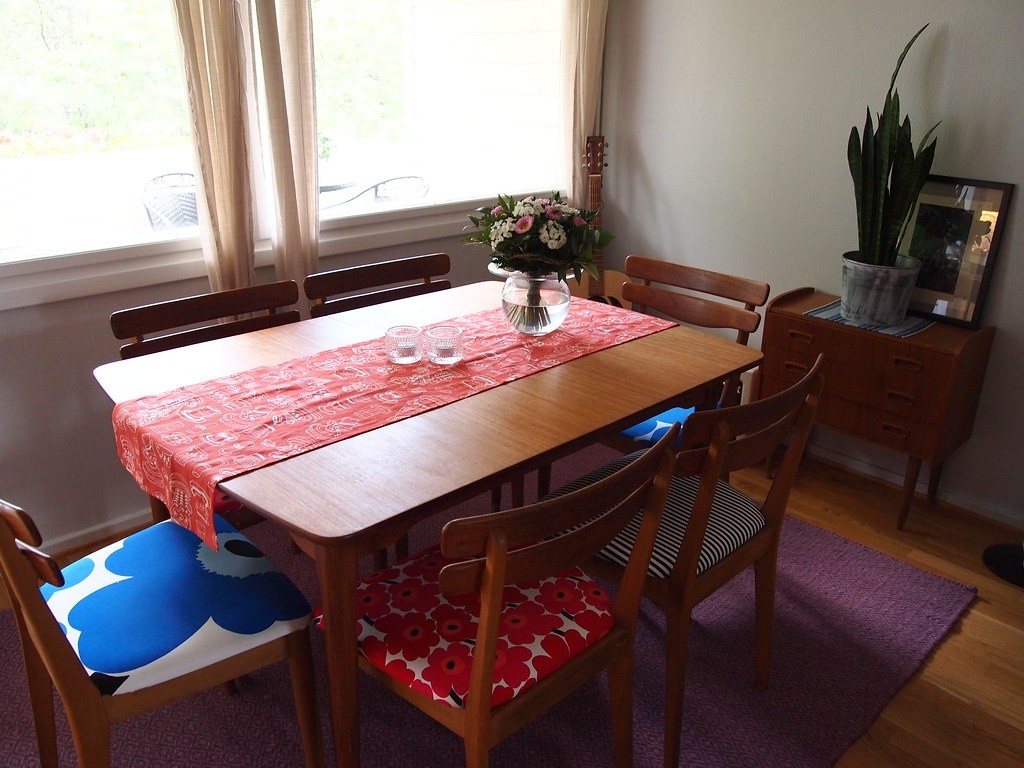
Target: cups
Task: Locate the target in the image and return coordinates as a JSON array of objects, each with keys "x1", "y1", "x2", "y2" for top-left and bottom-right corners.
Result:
[
  {"x1": 385, "y1": 324, "x2": 423, "y2": 364},
  {"x1": 426, "y1": 325, "x2": 464, "y2": 365}
]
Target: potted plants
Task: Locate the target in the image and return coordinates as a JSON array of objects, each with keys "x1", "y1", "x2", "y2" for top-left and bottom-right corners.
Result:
[{"x1": 839, "y1": 23, "x2": 953, "y2": 325}]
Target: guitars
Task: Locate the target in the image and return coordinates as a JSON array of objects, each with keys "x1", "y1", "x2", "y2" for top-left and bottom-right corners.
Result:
[{"x1": 564, "y1": 136, "x2": 633, "y2": 310}]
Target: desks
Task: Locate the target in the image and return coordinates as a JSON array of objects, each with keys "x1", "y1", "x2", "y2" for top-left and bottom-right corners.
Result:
[
  {"x1": 94, "y1": 281, "x2": 766, "y2": 767},
  {"x1": 314, "y1": 167, "x2": 356, "y2": 209}
]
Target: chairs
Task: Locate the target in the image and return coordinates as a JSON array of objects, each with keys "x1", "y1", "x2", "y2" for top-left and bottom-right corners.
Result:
[
  {"x1": 532, "y1": 353, "x2": 830, "y2": 767},
  {"x1": 303, "y1": 253, "x2": 505, "y2": 561},
  {"x1": 536, "y1": 252, "x2": 771, "y2": 499},
  {"x1": 0, "y1": 490, "x2": 318, "y2": 768},
  {"x1": 310, "y1": 422, "x2": 685, "y2": 768},
  {"x1": 320, "y1": 174, "x2": 431, "y2": 221},
  {"x1": 109, "y1": 278, "x2": 386, "y2": 574},
  {"x1": 141, "y1": 171, "x2": 200, "y2": 230}
]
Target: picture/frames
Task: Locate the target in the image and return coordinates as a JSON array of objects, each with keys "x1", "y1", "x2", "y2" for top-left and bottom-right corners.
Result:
[{"x1": 894, "y1": 173, "x2": 1016, "y2": 325}]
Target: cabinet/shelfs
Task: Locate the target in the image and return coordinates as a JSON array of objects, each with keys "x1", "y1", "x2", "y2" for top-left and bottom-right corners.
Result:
[{"x1": 749, "y1": 286, "x2": 994, "y2": 531}]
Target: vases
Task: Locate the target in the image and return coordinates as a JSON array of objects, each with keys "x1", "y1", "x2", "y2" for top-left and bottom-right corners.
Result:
[{"x1": 487, "y1": 260, "x2": 589, "y2": 336}]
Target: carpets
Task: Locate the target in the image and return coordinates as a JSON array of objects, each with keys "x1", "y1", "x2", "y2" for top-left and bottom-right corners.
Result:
[{"x1": 0, "y1": 492, "x2": 976, "y2": 768}]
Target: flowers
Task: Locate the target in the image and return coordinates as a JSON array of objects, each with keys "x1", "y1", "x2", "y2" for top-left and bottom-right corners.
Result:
[{"x1": 464, "y1": 188, "x2": 614, "y2": 274}]
